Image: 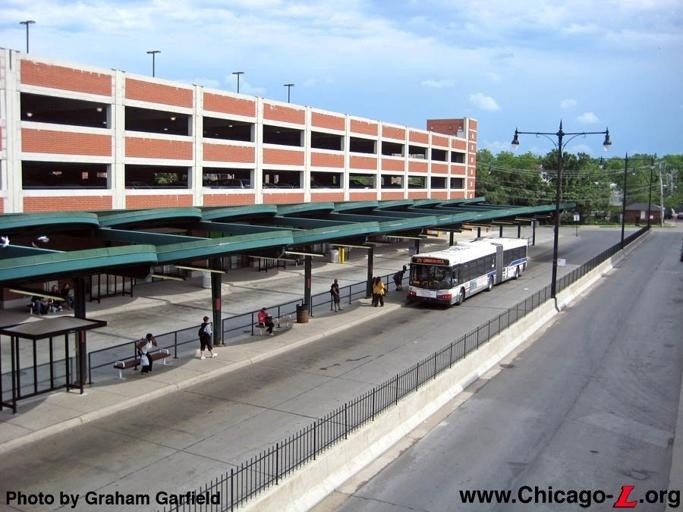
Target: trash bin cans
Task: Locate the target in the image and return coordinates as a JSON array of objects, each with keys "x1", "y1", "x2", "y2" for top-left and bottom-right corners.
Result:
[
  {"x1": 296, "y1": 304, "x2": 309, "y2": 323},
  {"x1": 330, "y1": 250, "x2": 339, "y2": 263}
]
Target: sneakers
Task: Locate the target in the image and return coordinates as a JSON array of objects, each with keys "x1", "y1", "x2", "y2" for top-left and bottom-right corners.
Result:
[{"x1": 201, "y1": 352, "x2": 218, "y2": 360}]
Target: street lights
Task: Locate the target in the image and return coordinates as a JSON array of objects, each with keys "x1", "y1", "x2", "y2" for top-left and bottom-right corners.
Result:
[
  {"x1": 511, "y1": 121, "x2": 613, "y2": 297},
  {"x1": 599, "y1": 154, "x2": 672, "y2": 250}
]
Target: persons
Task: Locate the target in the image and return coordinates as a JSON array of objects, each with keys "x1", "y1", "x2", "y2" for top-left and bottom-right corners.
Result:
[
  {"x1": 373, "y1": 277, "x2": 385, "y2": 307},
  {"x1": 138, "y1": 333, "x2": 158, "y2": 375},
  {"x1": 392, "y1": 271, "x2": 403, "y2": 291},
  {"x1": 30, "y1": 283, "x2": 74, "y2": 316},
  {"x1": 198, "y1": 316, "x2": 218, "y2": 360},
  {"x1": 258, "y1": 307, "x2": 275, "y2": 336},
  {"x1": 330, "y1": 279, "x2": 344, "y2": 312},
  {"x1": 371, "y1": 277, "x2": 376, "y2": 305}
]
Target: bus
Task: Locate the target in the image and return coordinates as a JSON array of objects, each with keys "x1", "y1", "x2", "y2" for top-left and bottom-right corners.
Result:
[{"x1": 408, "y1": 238, "x2": 529, "y2": 306}]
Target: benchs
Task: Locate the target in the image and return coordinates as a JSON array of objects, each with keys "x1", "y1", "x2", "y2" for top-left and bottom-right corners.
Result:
[
  {"x1": 254, "y1": 317, "x2": 290, "y2": 336},
  {"x1": 25, "y1": 304, "x2": 36, "y2": 314},
  {"x1": 113, "y1": 349, "x2": 171, "y2": 379}
]
